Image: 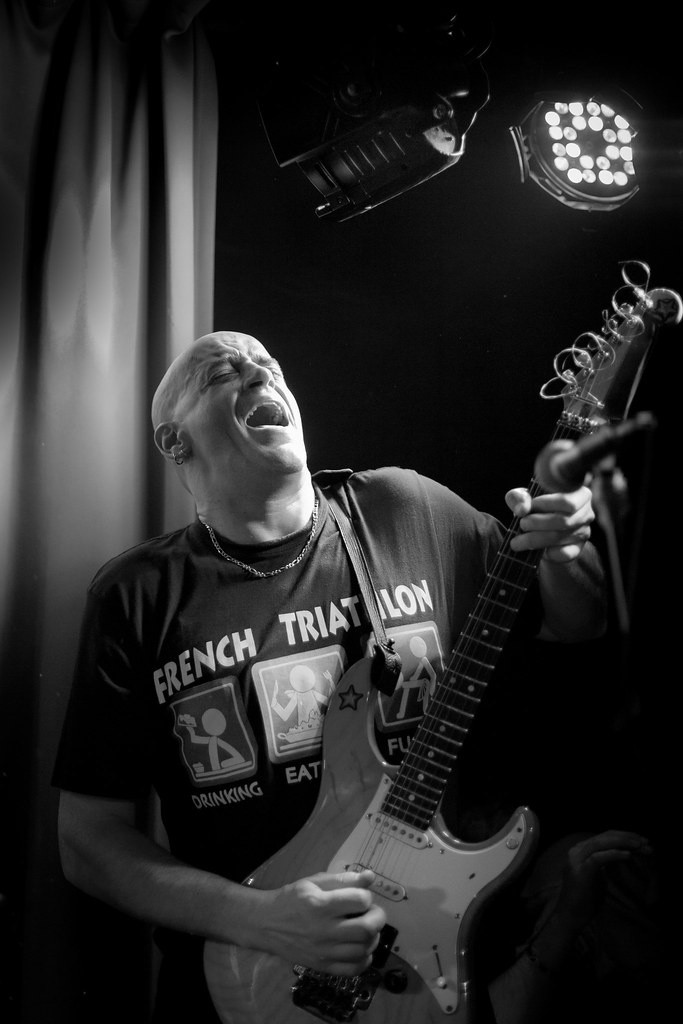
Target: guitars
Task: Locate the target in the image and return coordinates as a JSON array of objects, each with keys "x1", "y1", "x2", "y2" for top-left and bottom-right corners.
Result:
[{"x1": 179, "y1": 280, "x2": 683, "y2": 1024}]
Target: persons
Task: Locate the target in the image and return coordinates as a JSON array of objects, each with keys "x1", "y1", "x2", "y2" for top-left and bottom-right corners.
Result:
[{"x1": 49, "y1": 332, "x2": 599, "y2": 1023}]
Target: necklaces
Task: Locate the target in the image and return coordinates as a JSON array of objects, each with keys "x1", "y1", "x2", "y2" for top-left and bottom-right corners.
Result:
[{"x1": 203, "y1": 498, "x2": 320, "y2": 577}]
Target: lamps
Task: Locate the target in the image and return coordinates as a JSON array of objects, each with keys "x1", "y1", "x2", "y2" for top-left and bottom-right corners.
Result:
[
  {"x1": 256, "y1": 13, "x2": 494, "y2": 224},
  {"x1": 508, "y1": 68, "x2": 658, "y2": 214}
]
